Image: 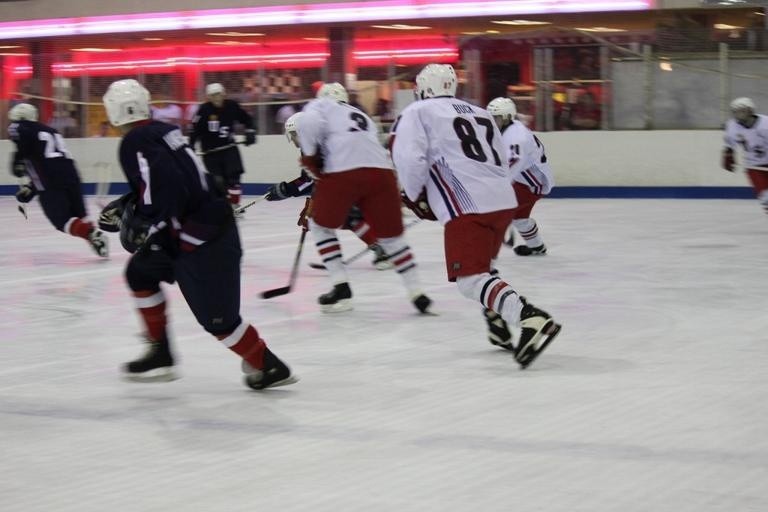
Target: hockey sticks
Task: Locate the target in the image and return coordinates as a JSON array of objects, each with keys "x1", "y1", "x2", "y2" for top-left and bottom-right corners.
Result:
[
  {"x1": 96, "y1": 166, "x2": 145, "y2": 246},
  {"x1": 259, "y1": 185, "x2": 314, "y2": 298},
  {"x1": 18, "y1": 165, "x2": 27, "y2": 219},
  {"x1": 308, "y1": 216, "x2": 422, "y2": 270}
]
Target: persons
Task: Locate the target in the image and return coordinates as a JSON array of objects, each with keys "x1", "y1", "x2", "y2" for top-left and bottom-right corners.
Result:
[
  {"x1": 565, "y1": 92, "x2": 601, "y2": 129},
  {"x1": 5, "y1": 101, "x2": 108, "y2": 259},
  {"x1": 272, "y1": 102, "x2": 303, "y2": 136},
  {"x1": 484, "y1": 96, "x2": 555, "y2": 255},
  {"x1": 90, "y1": 119, "x2": 112, "y2": 138},
  {"x1": 718, "y1": 95, "x2": 768, "y2": 214},
  {"x1": 60, "y1": 107, "x2": 81, "y2": 138},
  {"x1": 510, "y1": 97, "x2": 534, "y2": 129},
  {"x1": 282, "y1": 122, "x2": 389, "y2": 270},
  {"x1": 149, "y1": 92, "x2": 183, "y2": 128},
  {"x1": 97, "y1": 76, "x2": 291, "y2": 393},
  {"x1": 388, "y1": 61, "x2": 554, "y2": 364},
  {"x1": 187, "y1": 80, "x2": 258, "y2": 214},
  {"x1": 263, "y1": 80, "x2": 431, "y2": 314}
]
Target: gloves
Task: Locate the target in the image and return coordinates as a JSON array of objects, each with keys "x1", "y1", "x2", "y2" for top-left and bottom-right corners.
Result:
[
  {"x1": 724, "y1": 147, "x2": 735, "y2": 173},
  {"x1": 244, "y1": 133, "x2": 255, "y2": 146},
  {"x1": 12, "y1": 151, "x2": 27, "y2": 178},
  {"x1": 265, "y1": 181, "x2": 293, "y2": 201},
  {"x1": 97, "y1": 190, "x2": 136, "y2": 233},
  {"x1": 119, "y1": 197, "x2": 151, "y2": 254},
  {"x1": 15, "y1": 181, "x2": 37, "y2": 203}
]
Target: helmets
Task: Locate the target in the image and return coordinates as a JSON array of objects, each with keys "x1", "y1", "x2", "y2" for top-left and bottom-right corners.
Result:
[
  {"x1": 728, "y1": 97, "x2": 757, "y2": 112},
  {"x1": 7, "y1": 103, "x2": 39, "y2": 122},
  {"x1": 205, "y1": 83, "x2": 224, "y2": 96},
  {"x1": 416, "y1": 63, "x2": 458, "y2": 98},
  {"x1": 486, "y1": 97, "x2": 517, "y2": 120},
  {"x1": 315, "y1": 82, "x2": 349, "y2": 104},
  {"x1": 284, "y1": 112, "x2": 308, "y2": 148},
  {"x1": 102, "y1": 78, "x2": 152, "y2": 127}
]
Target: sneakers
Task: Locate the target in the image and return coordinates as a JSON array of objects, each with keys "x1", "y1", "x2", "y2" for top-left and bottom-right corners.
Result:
[
  {"x1": 413, "y1": 294, "x2": 430, "y2": 313},
  {"x1": 241, "y1": 347, "x2": 291, "y2": 390},
  {"x1": 513, "y1": 243, "x2": 547, "y2": 256},
  {"x1": 513, "y1": 299, "x2": 554, "y2": 364},
  {"x1": 127, "y1": 333, "x2": 175, "y2": 373},
  {"x1": 370, "y1": 242, "x2": 388, "y2": 264},
  {"x1": 483, "y1": 307, "x2": 512, "y2": 350},
  {"x1": 318, "y1": 282, "x2": 352, "y2": 305},
  {"x1": 88, "y1": 227, "x2": 106, "y2": 257}
]
